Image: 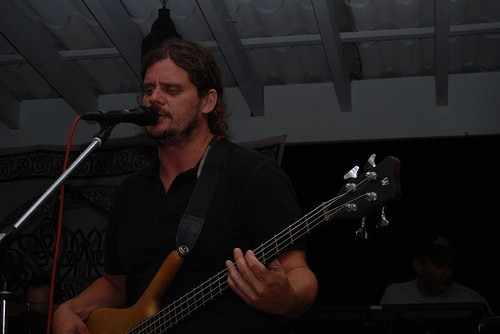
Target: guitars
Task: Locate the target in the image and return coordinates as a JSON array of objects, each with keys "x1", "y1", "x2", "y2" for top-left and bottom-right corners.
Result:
[{"x1": 80, "y1": 152, "x2": 402, "y2": 334}]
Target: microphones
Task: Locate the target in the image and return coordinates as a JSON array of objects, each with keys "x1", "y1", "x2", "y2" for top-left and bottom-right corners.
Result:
[{"x1": 79, "y1": 105, "x2": 159, "y2": 126}]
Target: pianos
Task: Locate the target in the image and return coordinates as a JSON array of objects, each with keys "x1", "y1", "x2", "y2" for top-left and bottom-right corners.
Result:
[{"x1": 279, "y1": 299, "x2": 495, "y2": 324}]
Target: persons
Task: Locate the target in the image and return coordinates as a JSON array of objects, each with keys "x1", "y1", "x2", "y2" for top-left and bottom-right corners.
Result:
[
  {"x1": 380, "y1": 235, "x2": 494, "y2": 317},
  {"x1": 52, "y1": 38, "x2": 318, "y2": 334}
]
